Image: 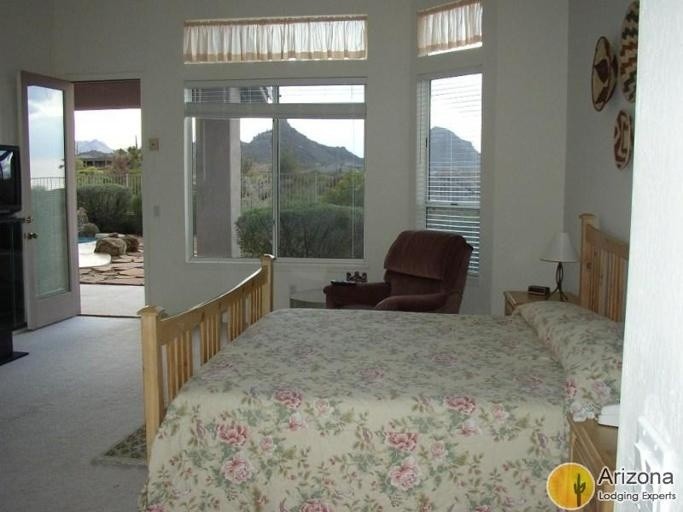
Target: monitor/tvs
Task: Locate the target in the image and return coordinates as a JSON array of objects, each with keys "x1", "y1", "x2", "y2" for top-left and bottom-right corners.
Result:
[{"x1": 0, "y1": 144, "x2": 22, "y2": 214}]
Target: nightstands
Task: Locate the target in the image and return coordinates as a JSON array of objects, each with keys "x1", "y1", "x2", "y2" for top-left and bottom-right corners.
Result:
[
  {"x1": 502, "y1": 289, "x2": 580, "y2": 316},
  {"x1": 565, "y1": 410, "x2": 618, "y2": 512}
]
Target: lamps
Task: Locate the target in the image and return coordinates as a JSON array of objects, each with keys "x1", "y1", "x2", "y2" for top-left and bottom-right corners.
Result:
[{"x1": 537, "y1": 231, "x2": 579, "y2": 301}]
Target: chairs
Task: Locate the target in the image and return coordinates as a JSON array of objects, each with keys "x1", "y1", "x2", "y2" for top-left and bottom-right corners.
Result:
[{"x1": 323, "y1": 229, "x2": 474, "y2": 314}]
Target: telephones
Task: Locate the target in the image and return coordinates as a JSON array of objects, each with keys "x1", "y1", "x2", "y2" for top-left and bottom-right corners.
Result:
[{"x1": 597, "y1": 404, "x2": 620, "y2": 427}]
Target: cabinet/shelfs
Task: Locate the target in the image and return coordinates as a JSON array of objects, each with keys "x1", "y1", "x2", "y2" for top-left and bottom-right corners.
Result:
[{"x1": 0, "y1": 216, "x2": 28, "y2": 331}]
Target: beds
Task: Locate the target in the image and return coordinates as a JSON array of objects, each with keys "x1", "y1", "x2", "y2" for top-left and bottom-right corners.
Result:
[
  {"x1": 288, "y1": 289, "x2": 327, "y2": 309},
  {"x1": 136, "y1": 213, "x2": 629, "y2": 512}
]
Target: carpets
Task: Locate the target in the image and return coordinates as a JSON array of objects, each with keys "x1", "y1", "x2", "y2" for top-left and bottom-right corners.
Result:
[{"x1": 90, "y1": 405, "x2": 168, "y2": 466}]
